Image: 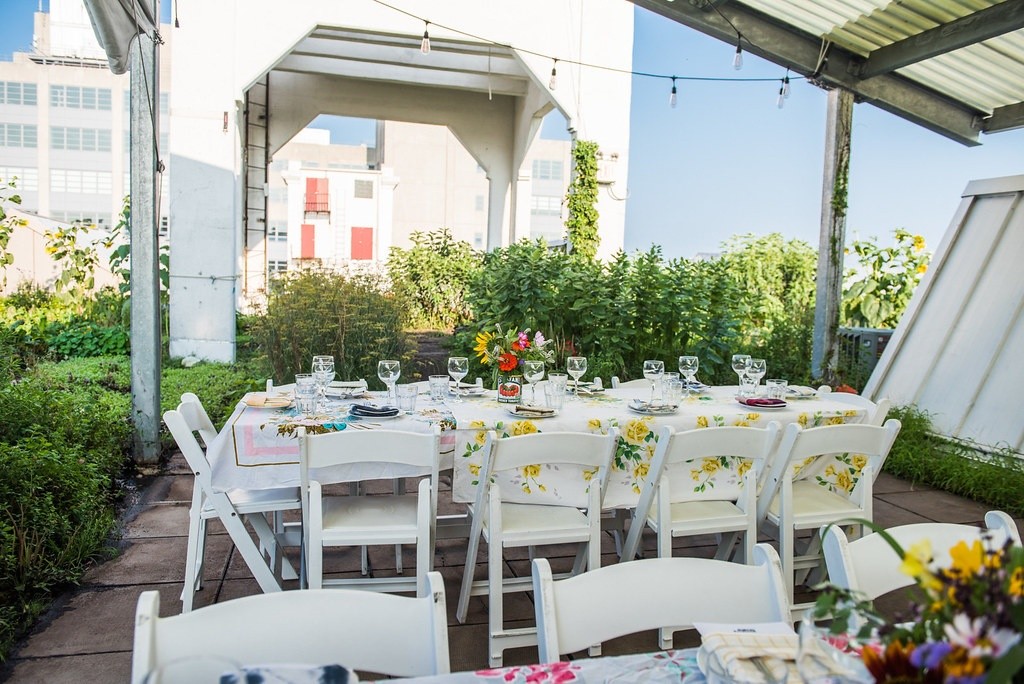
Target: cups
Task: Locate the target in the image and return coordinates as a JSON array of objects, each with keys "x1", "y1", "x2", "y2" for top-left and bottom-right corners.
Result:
[
  {"x1": 395, "y1": 384, "x2": 418, "y2": 415},
  {"x1": 429, "y1": 374, "x2": 450, "y2": 406},
  {"x1": 766, "y1": 379, "x2": 788, "y2": 401},
  {"x1": 293, "y1": 373, "x2": 318, "y2": 419},
  {"x1": 663, "y1": 381, "x2": 683, "y2": 408},
  {"x1": 796, "y1": 607, "x2": 887, "y2": 684},
  {"x1": 548, "y1": 373, "x2": 568, "y2": 404},
  {"x1": 661, "y1": 372, "x2": 680, "y2": 406},
  {"x1": 544, "y1": 383, "x2": 566, "y2": 415}
]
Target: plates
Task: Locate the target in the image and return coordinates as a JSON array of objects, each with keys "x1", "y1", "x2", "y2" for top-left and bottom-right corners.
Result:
[
  {"x1": 627, "y1": 406, "x2": 678, "y2": 415},
  {"x1": 738, "y1": 401, "x2": 787, "y2": 411},
  {"x1": 325, "y1": 392, "x2": 364, "y2": 400},
  {"x1": 347, "y1": 407, "x2": 406, "y2": 421},
  {"x1": 785, "y1": 394, "x2": 820, "y2": 400},
  {"x1": 696, "y1": 644, "x2": 711, "y2": 676},
  {"x1": 449, "y1": 388, "x2": 491, "y2": 397}
]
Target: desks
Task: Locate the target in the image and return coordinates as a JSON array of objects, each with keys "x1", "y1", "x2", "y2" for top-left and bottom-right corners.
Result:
[
  {"x1": 209, "y1": 381, "x2": 869, "y2": 534},
  {"x1": 358, "y1": 632, "x2": 836, "y2": 684}
]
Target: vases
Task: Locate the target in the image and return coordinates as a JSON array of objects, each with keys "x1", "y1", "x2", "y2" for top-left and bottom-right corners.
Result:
[{"x1": 497, "y1": 373, "x2": 524, "y2": 404}]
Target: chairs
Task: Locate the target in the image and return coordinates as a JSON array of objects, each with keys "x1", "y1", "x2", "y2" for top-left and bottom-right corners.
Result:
[
  {"x1": 816, "y1": 510, "x2": 1022, "y2": 623},
  {"x1": 620, "y1": 419, "x2": 783, "y2": 651},
  {"x1": 454, "y1": 426, "x2": 624, "y2": 670},
  {"x1": 762, "y1": 385, "x2": 892, "y2": 586},
  {"x1": 129, "y1": 567, "x2": 450, "y2": 684},
  {"x1": 731, "y1": 418, "x2": 909, "y2": 629},
  {"x1": 286, "y1": 422, "x2": 439, "y2": 596},
  {"x1": 531, "y1": 542, "x2": 796, "y2": 667},
  {"x1": 160, "y1": 391, "x2": 299, "y2": 609}
]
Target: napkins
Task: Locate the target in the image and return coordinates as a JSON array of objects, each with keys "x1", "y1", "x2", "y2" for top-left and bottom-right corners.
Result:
[
  {"x1": 734, "y1": 394, "x2": 788, "y2": 408},
  {"x1": 507, "y1": 401, "x2": 556, "y2": 417}
]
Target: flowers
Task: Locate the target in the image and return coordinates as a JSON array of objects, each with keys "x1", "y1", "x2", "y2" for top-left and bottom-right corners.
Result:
[
  {"x1": 474, "y1": 320, "x2": 556, "y2": 382},
  {"x1": 811, "y1": 518, "x2": 1024, "y2": 684}
]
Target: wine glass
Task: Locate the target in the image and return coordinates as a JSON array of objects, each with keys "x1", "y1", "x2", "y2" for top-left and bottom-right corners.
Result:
[
  {"x1": 642, "y1": 360, "x2": 664, "y2": 406},
  {"x1": 744, "y1": 358, "x2": 766, "y2": 398},
  {"x1": 523, "y1": 361, "x2": 545, "y2": 406},
  {"x1": 567, "y1": 357, "x2": 587, "y2": 401},
  {"x1": 678, "y1": 356, "x2": 699, "y2": 399},
  {"x1": 377, "y1": 360, "x2": 401, "y2": 407},
  {"x1": 311, "y1": 355, "x2": 336, "y2": 415},
  {"x1": 448, "y1": 356, "x2": 468, "y2": 405},
  {"x1": 731, "y1": 355, "x2": 752, "y2": 398}
]
